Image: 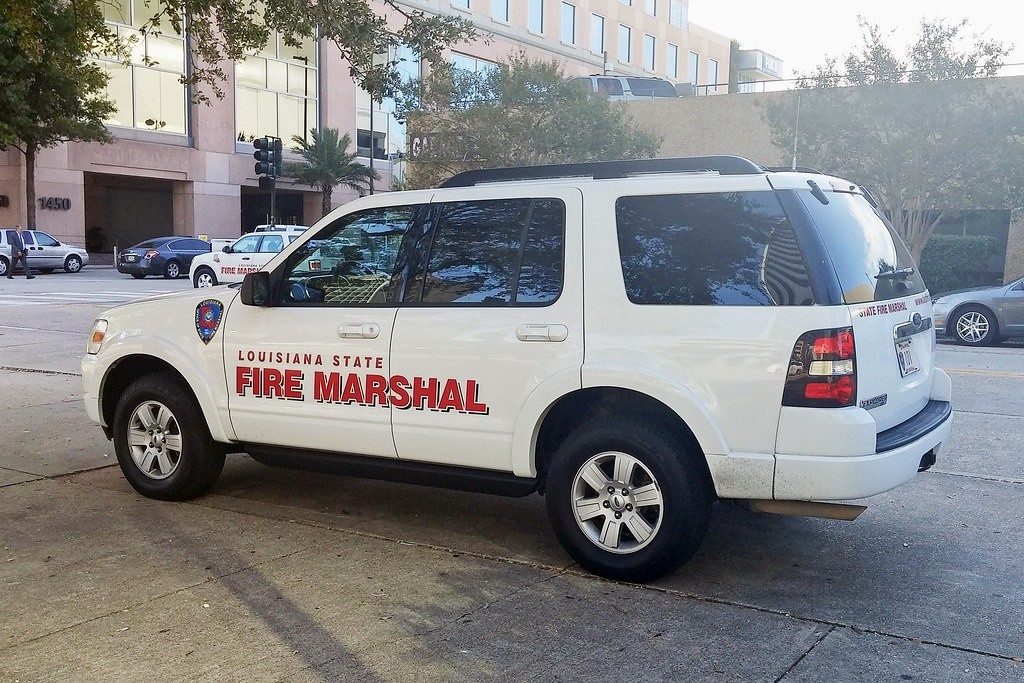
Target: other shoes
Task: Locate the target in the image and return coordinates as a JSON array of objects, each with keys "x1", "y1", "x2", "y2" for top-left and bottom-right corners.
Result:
[
  {"x1": 27, "y1": 276, "x2": 35, "y2": 279},
  {"x1": 7, "y1": 276, "x2": 14, "y2": 279}
]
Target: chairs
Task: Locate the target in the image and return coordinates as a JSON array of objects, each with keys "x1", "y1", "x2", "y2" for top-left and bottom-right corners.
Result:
[{"x1": 268, "y1": 241, "x2": 278, "y2": 252}]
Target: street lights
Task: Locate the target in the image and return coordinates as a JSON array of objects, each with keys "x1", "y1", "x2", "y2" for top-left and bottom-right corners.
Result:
[{"x1": 293, "y1": 54, "x2": 309, "y2": 152}]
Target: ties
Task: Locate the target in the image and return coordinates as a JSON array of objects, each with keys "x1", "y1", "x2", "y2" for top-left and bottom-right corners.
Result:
[{"x1": 19, "y1": 235, "x2": 23, "y2": 251}]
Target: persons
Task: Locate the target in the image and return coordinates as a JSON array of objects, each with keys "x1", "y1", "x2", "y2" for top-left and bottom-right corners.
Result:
[{"x1": 7, "y1": 224, "x2": 35, "y2": 279}]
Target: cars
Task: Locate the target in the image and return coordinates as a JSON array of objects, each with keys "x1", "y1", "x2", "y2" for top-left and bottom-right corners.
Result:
[
  {"x1": 930, "y1": 274, "x2": 1024, "y2": 349},
  {"x1": 117, "y1": 236, "x2": 212, "y2": 279},
  {"x1": 0, "y1": 228, "x2": 90, "y2": 277}
]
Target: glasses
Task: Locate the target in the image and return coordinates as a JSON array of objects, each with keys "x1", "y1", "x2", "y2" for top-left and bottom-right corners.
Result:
[{"x1": 20, "y1": 228, "x2": 23, "y2": 230}]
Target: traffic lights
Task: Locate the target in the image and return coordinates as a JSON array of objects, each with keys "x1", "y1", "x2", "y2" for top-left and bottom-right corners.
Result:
[
  {"x1": 268, "y1": 140, "x2": 283, "y2": 176},
  {"x1": 253, "y1": 137, "x2": 270, "y2": 175}
]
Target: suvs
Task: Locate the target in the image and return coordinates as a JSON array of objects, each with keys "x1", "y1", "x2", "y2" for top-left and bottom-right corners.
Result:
[
  {"x1": 187, "y1": 222, "x2": 345, "y2": 289},
  {"x1": 77, "y1": 153, "x2": 957, "y2": 588},
  {"x1": 553, "y1": 72, "x2": 678, "y2": 104}
]
